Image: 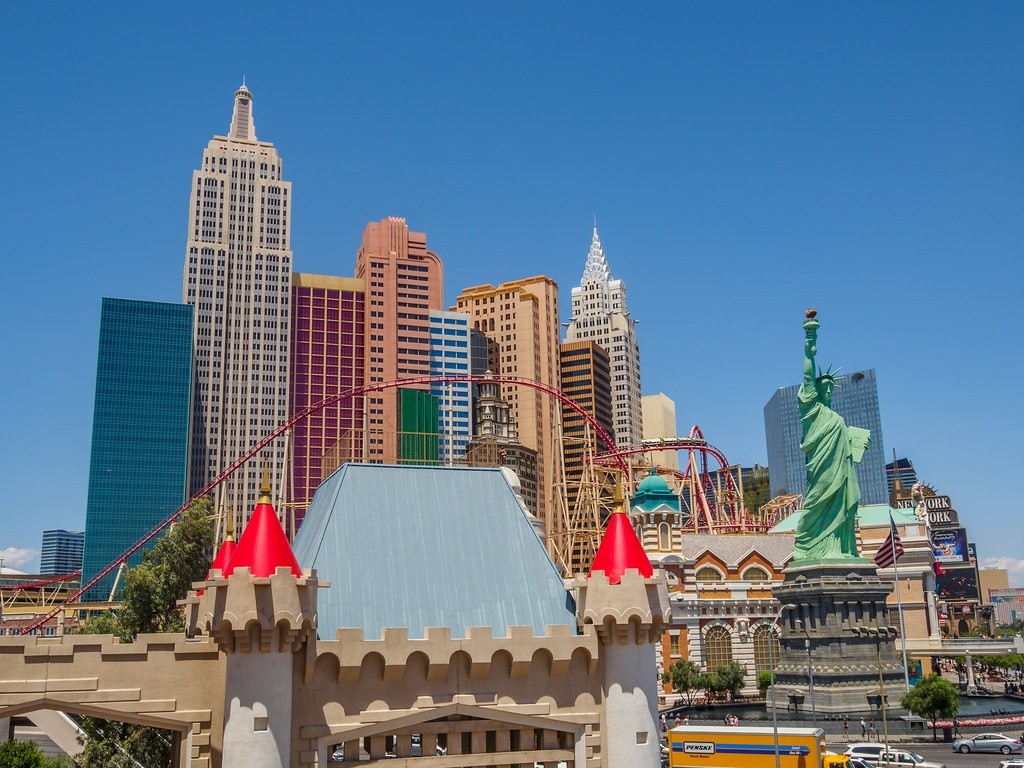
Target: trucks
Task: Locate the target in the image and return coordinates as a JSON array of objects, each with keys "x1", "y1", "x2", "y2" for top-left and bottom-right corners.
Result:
[{"x1": 668, "y1": 725, "x2": 855, "y2": 768}]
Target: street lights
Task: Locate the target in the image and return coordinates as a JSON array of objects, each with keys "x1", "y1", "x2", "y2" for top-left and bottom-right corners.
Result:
[
  {"x1": 852, "y1": 626, "x2": 898, "y2": 768},
  {"x1": 768, "y1": 604, "x2": 796, "y2": 768},
  {"x1": 796, "y1": 619, "x2": 816, "y2": 728}
]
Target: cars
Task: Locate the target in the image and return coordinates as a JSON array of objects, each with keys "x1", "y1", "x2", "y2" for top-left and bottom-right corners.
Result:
[
  {"x1": 844, "y1": 743, "x2": 923, "y2": 760},
  {"x1": 850, "y1": 757, "x2": 876, "y2": 768},
  {"x1": 659, "y1": 740, "x2": 669, "y2": 754},
  {"x1": 951, "y1": 734, "x2": 1022, "y2": 755},
  {"x1": 999, "y1": 760, "x2": 1024, "y2": 768}
]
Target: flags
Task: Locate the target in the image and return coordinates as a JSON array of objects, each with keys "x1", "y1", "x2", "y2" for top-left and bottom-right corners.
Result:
[{"x1": 874, "y1": 513, "x2": 905, "y2": 569}]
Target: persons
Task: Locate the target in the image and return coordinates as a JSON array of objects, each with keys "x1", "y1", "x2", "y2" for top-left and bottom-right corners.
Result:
[
  {"x1": 842, "y1": 720, "x2": 851, "y2": 740},
  {"x1": 952, "y1": 717, "x2": 963, "y2": 738},
  {"x1": 793, "y1": 326, "x2": 872, "y2": 562},
  {"x1": 724, "y1": 712, "x2": 740, "y2": 726},
  {"x1": 859, "y1": 717, "x2": 878, "y2": 740},
  {"x1": 661, "y1": 713, "x2": 690, "y2": 733},
  {"x1": 933, "y1": 540, "x2": 958, "y2": 558}
]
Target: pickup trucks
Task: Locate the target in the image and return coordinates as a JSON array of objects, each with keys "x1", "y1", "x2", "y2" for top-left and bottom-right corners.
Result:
[{"x1": 865, "y1": 749, "x2": 946, "y2": 768}]
